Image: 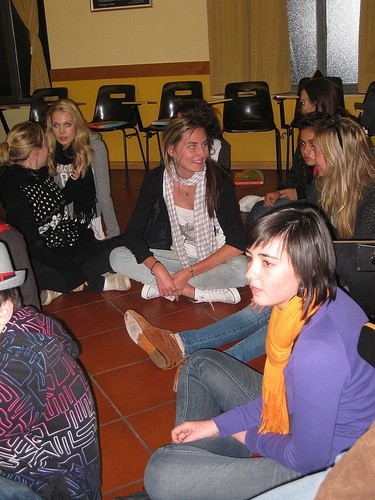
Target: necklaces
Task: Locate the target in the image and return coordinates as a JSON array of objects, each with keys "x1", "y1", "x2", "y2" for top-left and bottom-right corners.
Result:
[{"x1": 180, "y1": 186, "x2": 195, "y2": 196}]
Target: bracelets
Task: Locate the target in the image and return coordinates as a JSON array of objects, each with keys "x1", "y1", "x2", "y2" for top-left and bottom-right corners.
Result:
[
  {"x1": 191, "y1": 265, "x2": 194, "y2": 277},
  {"x1": 151, "y1": 261, "x2": 160, "y2": 275}
]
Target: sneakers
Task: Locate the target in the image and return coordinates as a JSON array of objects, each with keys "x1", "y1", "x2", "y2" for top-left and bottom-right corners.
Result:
[
  {"x1": 140, "y1": 270, "x2": 175, "y2": 300},
  {"x1": 193, "y1": 288, "x2": 241, "y2": 304}
]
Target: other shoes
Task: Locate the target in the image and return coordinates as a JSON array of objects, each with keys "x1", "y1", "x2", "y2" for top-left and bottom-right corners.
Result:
[
  {"x1": 104, "y1": 274, "x2": 131, "y2": 291},
  {"x1": 123, "y1": 309, "x2": 182, "y2": 369},
  {"x1": 238, "y1": 195, "x2": 265, "y2": 213},
  {"x1": 40, "y1": 289, "x2": 58, "y2": 305}
]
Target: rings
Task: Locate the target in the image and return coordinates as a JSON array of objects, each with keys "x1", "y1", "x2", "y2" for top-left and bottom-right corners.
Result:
[
  {"x1": 172, "y1": 290, "x2": 176, "y2": 292},
  {"x1": 284, "y1": 195, "x2": 286, "y2": 197}
]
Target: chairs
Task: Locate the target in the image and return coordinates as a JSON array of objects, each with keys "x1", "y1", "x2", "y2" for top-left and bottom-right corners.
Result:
[
  {"x1": 28, "y1": 88, "x2": 69, "y2": 123},
  {"x1": 88, "y1": 81, "x2": 282, "y2": 192}
]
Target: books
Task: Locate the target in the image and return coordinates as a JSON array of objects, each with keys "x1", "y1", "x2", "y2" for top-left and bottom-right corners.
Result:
[{"x1": 234, "y1": 172, "x2": 264, "y2": 185}]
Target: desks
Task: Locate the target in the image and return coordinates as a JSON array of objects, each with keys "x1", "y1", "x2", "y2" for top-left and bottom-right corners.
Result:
[{"x1": 274, "y1": 92, "x2": 301, "y2": 180}]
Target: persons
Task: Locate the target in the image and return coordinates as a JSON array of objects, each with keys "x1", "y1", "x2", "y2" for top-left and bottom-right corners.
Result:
[
  {"x1": 105, "y1": 119, "x2": 250, "y2": 305},
  {"x1": 176, "y1": 99, "x2": 232, "y2": 171},
  {"x1": 0, "y1": 221, "x2": 41, "y2": 311},
  {"x1": 116, "y1": 207, "x2": 375, "y2": 500},
  {"x1": 124, "y1": 107, "x2": 375, "y2": 393},
  {"x1": 248, "y1": 78, "x2": 357, "y2": 228},
  {"x1": 249, "y1": 319, "x2": 375, "y2": 500},
  {"x1": 0, "y1": 120, "x2": 132, "y2": 305},
  {"x1": 0, "y1": 242, "x2": 103, "y2": 500},
  {"x1": 45, "y1": 99, "x2": 119, "y2": 238},
  {"x1": 359, "y1": 81, "x2": 375, "y2": 147}
]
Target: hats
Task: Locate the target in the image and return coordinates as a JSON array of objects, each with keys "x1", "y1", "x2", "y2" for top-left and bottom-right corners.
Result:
[{"x1": 0, "y1": 242, "x2": 25, "y2": 291}]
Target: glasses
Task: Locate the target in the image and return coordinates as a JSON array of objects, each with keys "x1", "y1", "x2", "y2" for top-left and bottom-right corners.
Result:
[{"x1": 327, "y1": 113, "x2": 343, "y2": 148}]
